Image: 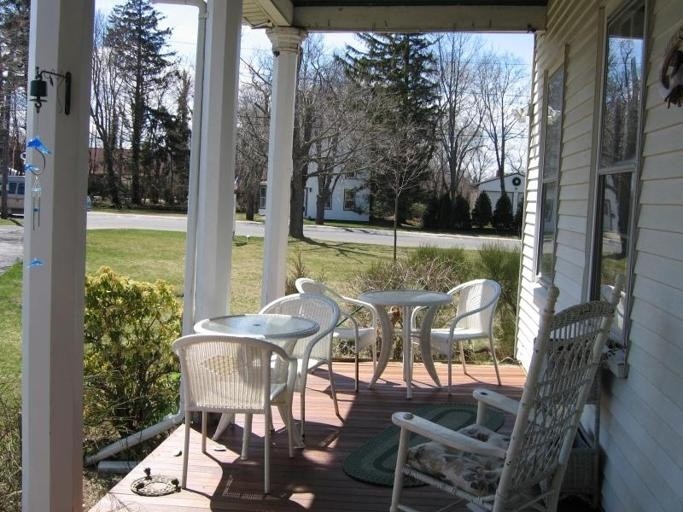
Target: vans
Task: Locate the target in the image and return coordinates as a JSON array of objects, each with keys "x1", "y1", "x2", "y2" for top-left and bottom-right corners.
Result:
[{"x1": 0, "y1": 176, "x2": 29, "y2": 215}]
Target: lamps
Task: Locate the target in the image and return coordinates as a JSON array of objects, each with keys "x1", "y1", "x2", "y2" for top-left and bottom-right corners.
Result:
[{"x1": 27, "y1": 65, "x2": 70, "y2": 116}]
